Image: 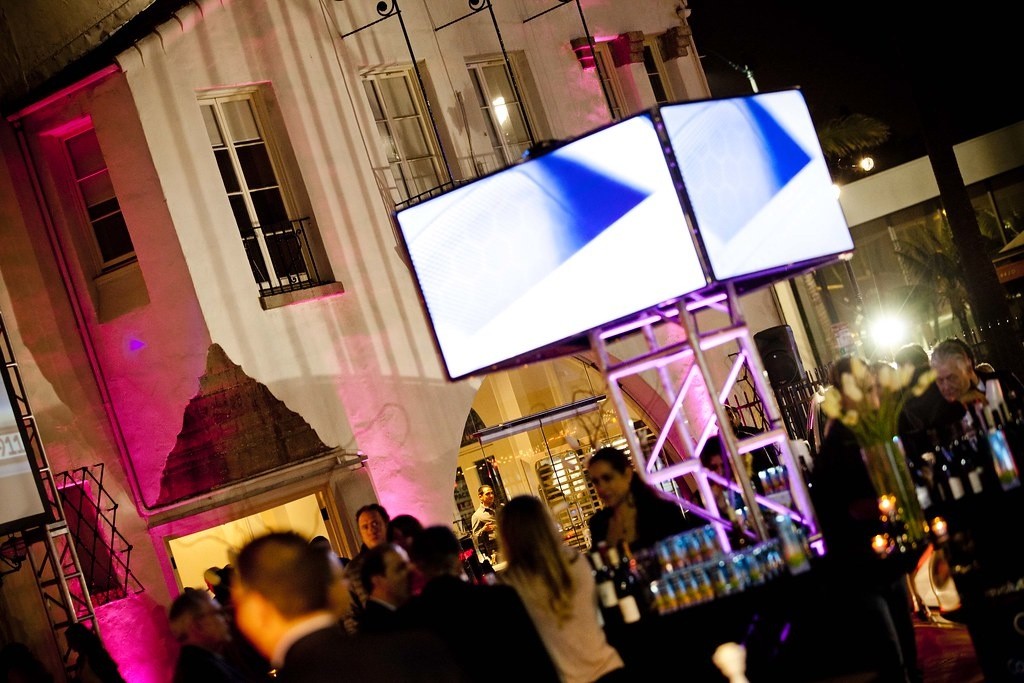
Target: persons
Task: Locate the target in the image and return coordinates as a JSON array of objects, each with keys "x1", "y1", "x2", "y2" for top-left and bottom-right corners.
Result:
[
  {"x1": 64, "y1": 623, "x2": 127, "y2": 683},
  {"x1": 725, "y1": 404, "x2": 780, "y2": 495},
  {"x1": 470, "y1": 485, "x2": 496, "y2": 535},
  {"x1": 808, "y1": 338, "x2": 1011, "y2": 531},
  {"x1": 687, "y1": 435, "x2": 744, "y2": 525},
  {"x1": 496, "y1": 494, "x2": 624, "y2": 683},
  {"x1": 170, "y1": 503, "x2": 563, "y2": 683},
  {"x1": 587, "y1": 447, "x2": 682, "y2": 554}
]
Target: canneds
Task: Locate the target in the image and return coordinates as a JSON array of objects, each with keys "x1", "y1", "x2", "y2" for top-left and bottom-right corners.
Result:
[{"x1": 648, "y1": 525, "x2": 786, "y2": 615}]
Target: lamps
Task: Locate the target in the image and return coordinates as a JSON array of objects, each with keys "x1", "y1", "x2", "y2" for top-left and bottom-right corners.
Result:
[{"x1": 838, "y1": 153, "x2": 874, "y2": 173}]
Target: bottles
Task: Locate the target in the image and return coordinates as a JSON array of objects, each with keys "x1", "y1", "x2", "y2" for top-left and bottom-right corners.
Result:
[
  {"x1": 587, "y1": 538, "x2": 660, "y2": 625},
  {"x1": 901, "y1": 388, "x2": 1023, "y2": 519}
]
